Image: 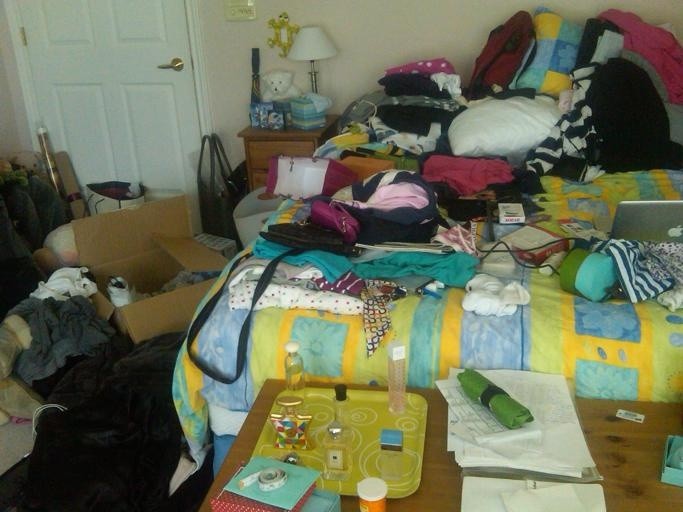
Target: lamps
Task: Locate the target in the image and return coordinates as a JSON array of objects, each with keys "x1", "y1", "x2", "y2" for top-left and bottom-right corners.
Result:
[{"x1": 287, "y1": 23, "x2": 337, "y2": 96}]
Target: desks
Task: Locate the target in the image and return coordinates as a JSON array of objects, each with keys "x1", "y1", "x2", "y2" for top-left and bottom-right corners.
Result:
[{"x1": 199, "y1": 377, "x2": 683, "y2": 512}]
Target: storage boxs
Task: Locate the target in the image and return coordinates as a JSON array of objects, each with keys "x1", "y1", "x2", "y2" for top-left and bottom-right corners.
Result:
[{"x1": 71, "y1": 194, "x2": 230, "y2": 348}]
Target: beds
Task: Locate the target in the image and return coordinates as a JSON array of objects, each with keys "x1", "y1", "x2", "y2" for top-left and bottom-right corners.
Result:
[{"x1": 172, "y1": 102, "x2": 683, "y2": 487}]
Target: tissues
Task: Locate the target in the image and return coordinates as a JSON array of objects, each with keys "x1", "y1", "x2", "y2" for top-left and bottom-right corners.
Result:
[{"x1": 288, "y1": 92, "x2": 333, "y2": 131}]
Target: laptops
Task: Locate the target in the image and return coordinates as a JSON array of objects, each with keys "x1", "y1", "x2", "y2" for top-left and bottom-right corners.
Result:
[{"x1": 592, "y1": 199, "x2": 683, "y2": 243}]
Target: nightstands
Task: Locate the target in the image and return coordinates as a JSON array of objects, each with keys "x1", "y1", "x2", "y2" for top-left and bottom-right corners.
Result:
[{"x1": 237, "y1": 112, "x2": 336, "y2": 191}]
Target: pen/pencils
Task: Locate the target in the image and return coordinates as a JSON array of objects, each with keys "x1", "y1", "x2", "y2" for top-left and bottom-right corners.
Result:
[{"x1": 421, "y1": 286, "x2": 443, "y2": 299}]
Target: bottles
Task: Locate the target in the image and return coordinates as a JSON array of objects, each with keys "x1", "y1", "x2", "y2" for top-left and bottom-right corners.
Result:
[
  {"x1": 332, "y1": 383, "x2": 351, "y2": 436},
  {"x1": 285, "y1": 342, "x2": 304, "y2": 395}
]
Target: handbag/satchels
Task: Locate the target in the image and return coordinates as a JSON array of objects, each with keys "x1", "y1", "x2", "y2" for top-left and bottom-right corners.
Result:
[
  {"x1": 465, "y1": 11, "x2": 536, "y2": 100},
  {"x1": 266, "y1": 153, "x2": 357, "y2": 200},
  {"x1": 85, "y1": 181, "x2": 145, "y2": 216},
  {"x1": 200, "y1": 160, "x2": 248, "y2": 252},
  {"x1": 259, "y1": 220, "x2": 352, "y2": 257},
  {"x1": 311, "y1": 200, "x2": 360, "y2": 243}
]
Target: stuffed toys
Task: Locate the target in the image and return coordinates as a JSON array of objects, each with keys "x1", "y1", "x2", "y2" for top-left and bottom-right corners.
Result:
[
  {"x1": 262, "y1": 72, "x2": 301, "y2": 102},
  {"x1": 7, "y1": 151, "x2": 44, "y2": 180}
]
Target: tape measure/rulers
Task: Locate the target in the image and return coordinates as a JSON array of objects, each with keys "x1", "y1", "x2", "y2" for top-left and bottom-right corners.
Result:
[{"x1": 239, "y1": 468, "x2": 288, "y2": 491}]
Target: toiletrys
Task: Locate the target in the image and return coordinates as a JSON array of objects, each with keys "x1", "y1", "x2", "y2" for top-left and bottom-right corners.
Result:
[
  {"x1": 285, "y1": 343, "x2": 307, "y2": 400},
  {"x1": 387, "y1": 343, "x2": 407, "y2": 413}
]
